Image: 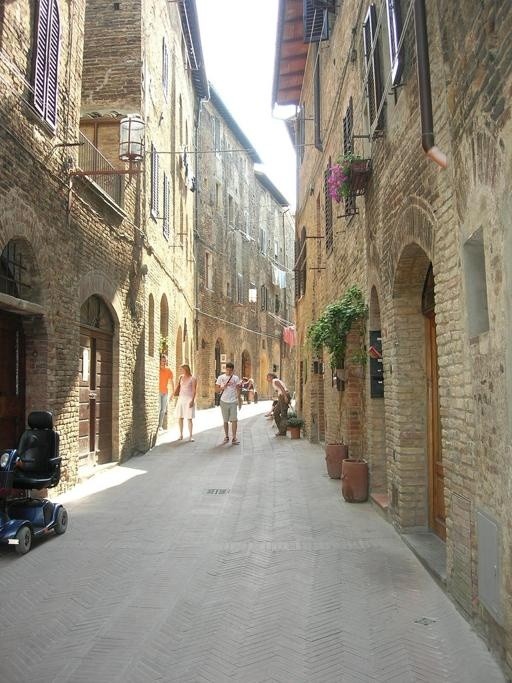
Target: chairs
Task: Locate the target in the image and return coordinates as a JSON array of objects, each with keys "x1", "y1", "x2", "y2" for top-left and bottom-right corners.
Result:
[{"x1": 12, "y1": 410, "x2": 60, "y2": 490}]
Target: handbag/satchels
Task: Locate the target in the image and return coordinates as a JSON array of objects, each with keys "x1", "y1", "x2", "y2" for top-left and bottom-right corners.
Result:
[
  {"x1": 215, "y1": 392, "x2": 222, "y2": 406},
  {"x1": 174, "y1": 375, "x2": 183, "y2": 396}
]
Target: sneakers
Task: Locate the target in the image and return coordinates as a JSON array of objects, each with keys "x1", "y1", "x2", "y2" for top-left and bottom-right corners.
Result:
[
  {"x1": 224, "y1": 436, "x2": 240, "y2": 444},
  {"x1": 178, "y1": 435, "x2": 195, "y2": 442}
]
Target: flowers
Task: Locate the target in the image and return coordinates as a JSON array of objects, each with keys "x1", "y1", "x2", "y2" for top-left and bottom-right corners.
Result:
[{"x1": 327, "y1": 151, "x2": 365, "y2": 204}]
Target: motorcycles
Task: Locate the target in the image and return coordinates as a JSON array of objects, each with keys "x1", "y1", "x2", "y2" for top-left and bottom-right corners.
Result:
[{"x1": 1, "y1": 410, "x2": 68, "y2": 554}]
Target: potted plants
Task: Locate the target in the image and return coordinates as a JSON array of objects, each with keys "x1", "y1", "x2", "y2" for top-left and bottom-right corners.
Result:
[{"x1": 287, "y1": 417, "x2": 305, "y2": 439}]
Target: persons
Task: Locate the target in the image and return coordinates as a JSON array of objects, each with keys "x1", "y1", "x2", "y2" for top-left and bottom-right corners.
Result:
[
  {"x1": 242, "y1": 376, "x2": 252, "y2": 389},
  {"x1": 158, "y1": 354, "x2": 175, "y2": 431},
  {"x1": 266, "y1": 373, "x2": 291, "y2": 436},
  {"x1": 215, "y1": 363, "x2": 243, "y2": 445},
  {"x1": 173, "y1": 364, "x2": 198, "y2": 442}
]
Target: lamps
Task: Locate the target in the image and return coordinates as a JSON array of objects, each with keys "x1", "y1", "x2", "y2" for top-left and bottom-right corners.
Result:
[{"x1": 66, "y1": 114, "x2": 144, "y2": 212}]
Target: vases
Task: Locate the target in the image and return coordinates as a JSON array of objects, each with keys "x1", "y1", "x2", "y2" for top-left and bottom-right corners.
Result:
[{"x1": 325, "y1": 443, "x2": 369, "y2": 503}]
